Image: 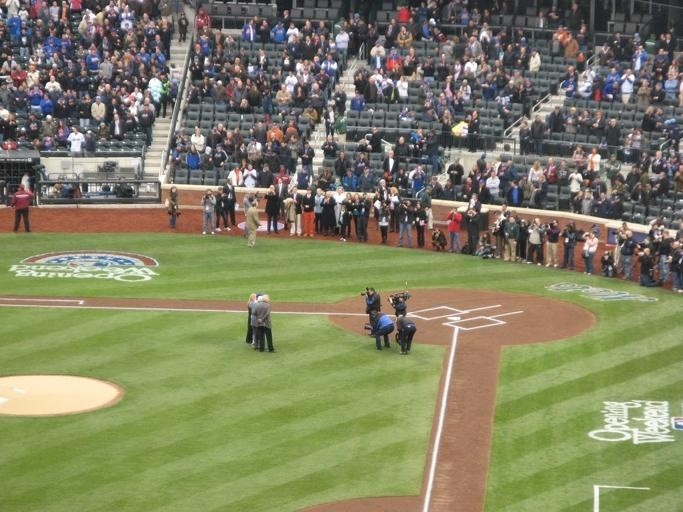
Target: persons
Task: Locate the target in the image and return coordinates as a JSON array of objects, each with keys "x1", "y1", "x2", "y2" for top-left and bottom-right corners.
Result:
[
  {"x1": 368, "y1": 307, "x2": 394, "y2": 350},
  {"x1": 246, "y1": 292, "x2": 277, "y2": 353},
  {"x1": 186, "y1": 1, "x2": 682, "y2": 296},
  {"x1": 388, "y1": 295, "x2": 409, "y2": 318},
  {"x1": 1, "y1": 1, "x2": 190, "y2": 232},
  {"x1": 392, "y1": 313, "x2": 416, "y2": 356},
  {"x1": 361, "y1": 286, "x2": 384, "y2": 330}
]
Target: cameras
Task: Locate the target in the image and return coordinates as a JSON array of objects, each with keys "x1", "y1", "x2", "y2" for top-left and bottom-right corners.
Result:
[
  {"x1": 364, "y1": 323, "x2": 373, "y2": 335},
  {"x1": 361, "y1": 288, "x2": 370, "y2": 298}
]
